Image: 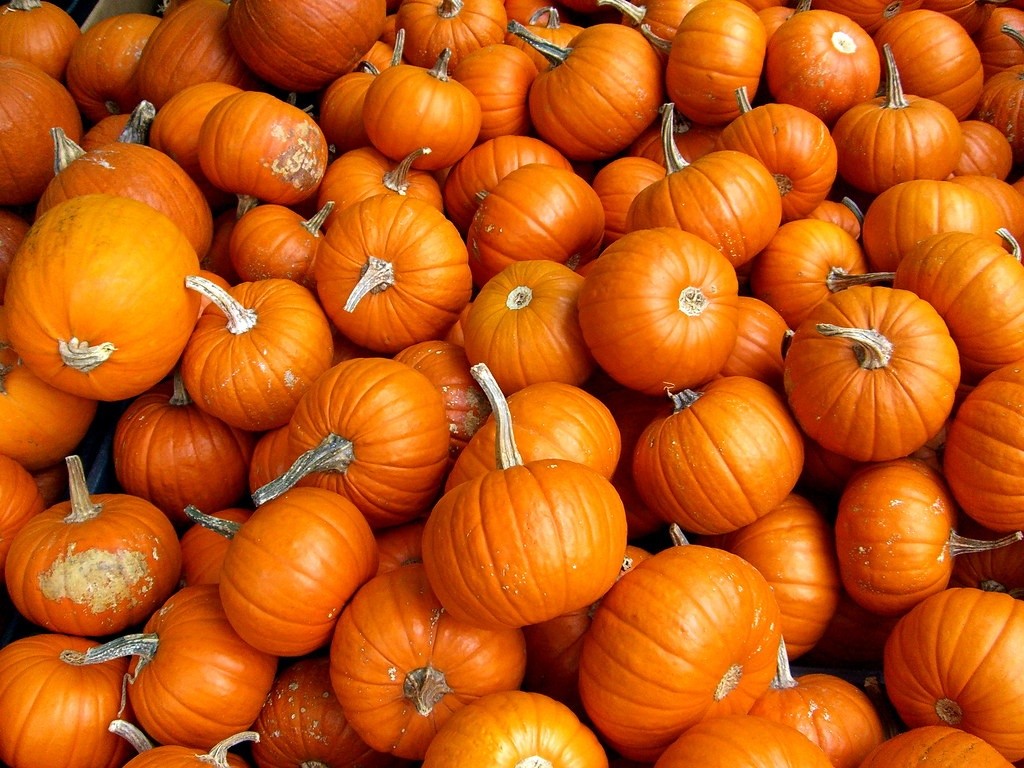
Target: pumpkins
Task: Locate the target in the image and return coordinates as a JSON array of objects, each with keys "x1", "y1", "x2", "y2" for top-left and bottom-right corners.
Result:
[{"x1": 0, "y1": 1, "x2": 1024, "y2": 768}]
